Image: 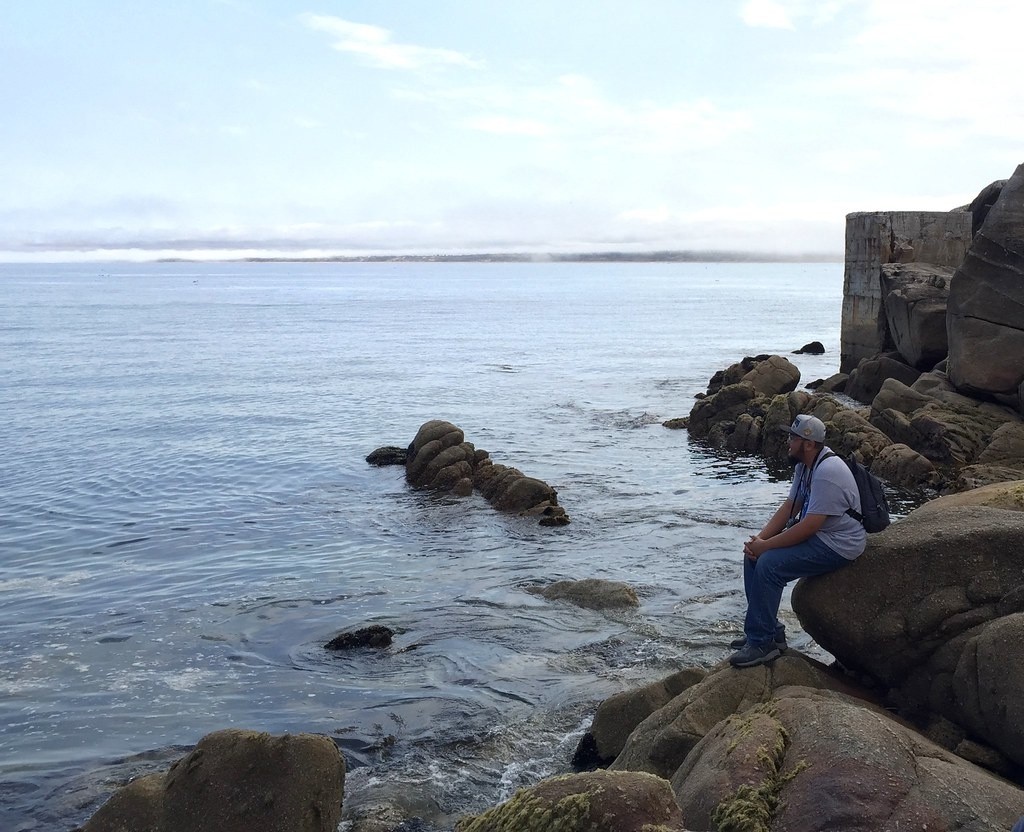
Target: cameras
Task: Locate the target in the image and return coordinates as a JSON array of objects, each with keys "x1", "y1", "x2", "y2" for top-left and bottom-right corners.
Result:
[{"x1": 786, "y1": 517, "x2": 801, "y2": 529}]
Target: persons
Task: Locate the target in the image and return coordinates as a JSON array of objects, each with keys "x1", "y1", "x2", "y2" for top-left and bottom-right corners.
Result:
[{"x1": 729, "y1": 413, "x2": 867, "y2": 668}]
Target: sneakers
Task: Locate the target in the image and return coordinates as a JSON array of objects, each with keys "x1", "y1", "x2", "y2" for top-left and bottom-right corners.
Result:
[
  {"x1": 728, "y1": 637, "x2": 781, "y2": 668},
  {"x1": 730, "y1": 630, "x2": 788, "y2": 651}
]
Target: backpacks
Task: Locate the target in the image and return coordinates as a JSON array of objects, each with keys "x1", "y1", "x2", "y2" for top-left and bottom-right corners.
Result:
[{"x1": 815, "y1": 451, "x2": 891, "y2": 533}]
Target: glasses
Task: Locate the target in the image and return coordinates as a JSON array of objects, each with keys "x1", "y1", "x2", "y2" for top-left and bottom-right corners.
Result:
[{"x1": 788, "y1": 433, "x2": 806, "y2": 442}]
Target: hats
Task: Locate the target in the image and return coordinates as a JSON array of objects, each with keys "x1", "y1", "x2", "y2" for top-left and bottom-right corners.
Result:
[{"x1": 779, "y1": 414, "x2": 827, "y2": 444}]
